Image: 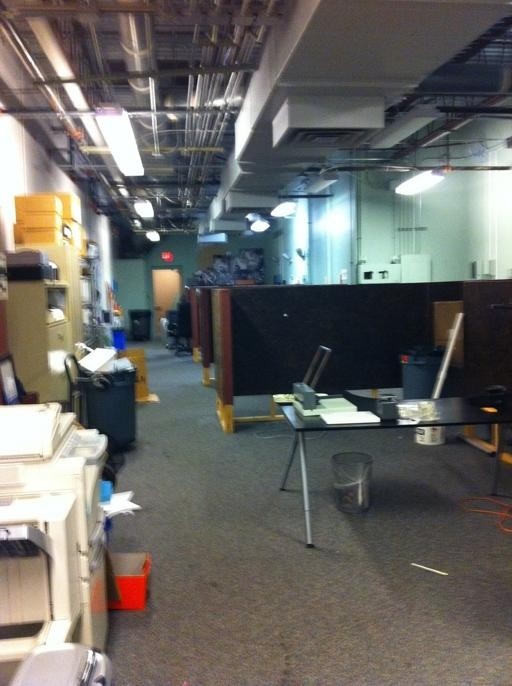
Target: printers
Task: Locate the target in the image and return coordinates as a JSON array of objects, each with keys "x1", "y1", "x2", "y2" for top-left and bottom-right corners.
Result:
[{"x1": 0, "y1": 403, "x2": 111, "y2": 686}]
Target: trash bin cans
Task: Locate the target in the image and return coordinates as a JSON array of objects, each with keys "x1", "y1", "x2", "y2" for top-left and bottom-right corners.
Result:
[
  {"x1": 76, "y1": 372, "x2": 137, "y2": 452},
  {"x1": 399, "y1": 345, "x2": 444, "y2": 399},
  {"x1": 330, "y1": 452, "x2": 373, "y2": 514},
  {"x1": 130, "y1": 310, "x2": 150, "y2": 341}
]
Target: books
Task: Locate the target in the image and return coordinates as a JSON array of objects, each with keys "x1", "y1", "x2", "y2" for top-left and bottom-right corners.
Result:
[{"x1": 321, "y1": 408, "x2": 382, "y2": 425}]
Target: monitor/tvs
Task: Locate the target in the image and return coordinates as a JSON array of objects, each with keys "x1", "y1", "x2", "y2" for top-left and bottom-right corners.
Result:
[{"x1": 0, "y1": 354, "x2": 20, "y2": 405}]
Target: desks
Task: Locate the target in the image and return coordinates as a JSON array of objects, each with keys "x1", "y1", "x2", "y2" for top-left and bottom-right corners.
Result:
[{"x1": 272, "y1": 382, "x2": 512, "y2": 549}]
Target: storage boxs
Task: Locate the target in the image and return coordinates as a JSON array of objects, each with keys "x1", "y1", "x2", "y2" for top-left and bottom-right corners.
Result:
[
  {"x1": 104, "y1": 549, "x2": 152, "y2": 611},
  {"x1": 12, "y1": 190, "x2": 88, "y2": 251},
  {"x1": 116, "y1": 348, "x2": 148, "y2": 401}
]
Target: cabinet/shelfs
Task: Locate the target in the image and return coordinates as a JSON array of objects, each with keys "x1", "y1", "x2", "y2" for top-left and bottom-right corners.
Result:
[{"x1": 6, "y1": 247, "x2": 102, "y2": 408}]
